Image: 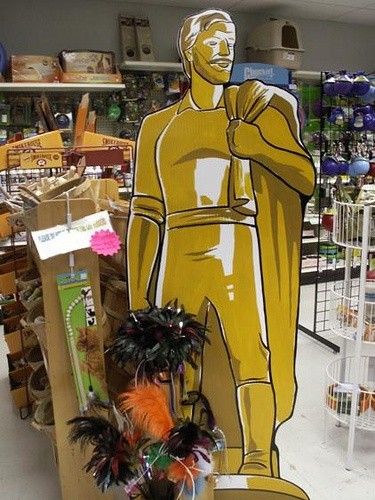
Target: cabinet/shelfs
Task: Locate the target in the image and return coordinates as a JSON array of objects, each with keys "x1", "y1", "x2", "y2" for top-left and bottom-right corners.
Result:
[
  {"x1": 291, "y1": 72, "x2": 375, "y2": 276},
  {"x1": 323, "y1": 183, "x2": 375, "y2": 474}
]
